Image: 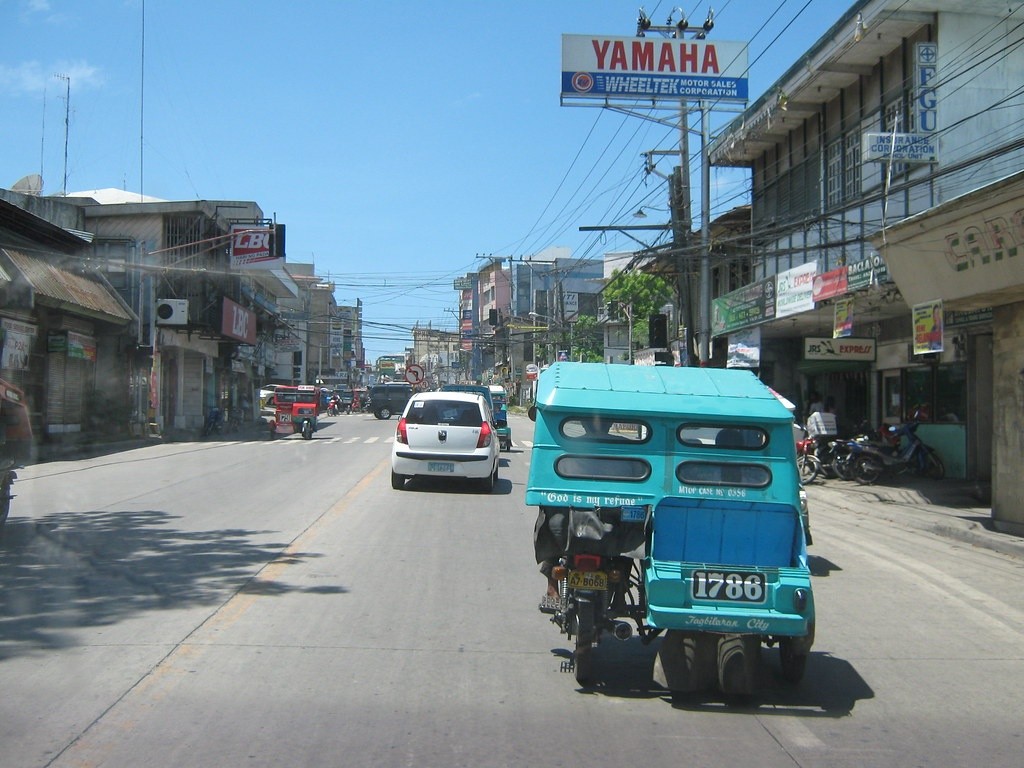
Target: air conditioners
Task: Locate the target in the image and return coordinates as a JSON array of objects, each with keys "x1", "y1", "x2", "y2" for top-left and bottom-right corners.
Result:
[{"x1": 156, "y1": 298, "x2": 190, "y2": 325}]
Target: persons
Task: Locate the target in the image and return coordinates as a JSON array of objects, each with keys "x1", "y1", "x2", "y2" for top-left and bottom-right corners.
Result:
[
  {"x1": 809, "y1": 390, "x2": 824, "y2": 415},
  {"x1": 327, "y1": 391, "x2": 342, "y2": 409},
  {"x1": 538, "y1": 416, "x2": 649, "y2": 614}
]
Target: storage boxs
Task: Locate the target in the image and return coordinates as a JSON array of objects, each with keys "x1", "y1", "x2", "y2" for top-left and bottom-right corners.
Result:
[{"x1": 807, "y1": 412, "x2": 838, "y2": 437}]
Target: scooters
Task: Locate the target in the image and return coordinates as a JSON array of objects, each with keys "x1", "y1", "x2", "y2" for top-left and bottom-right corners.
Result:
[{"x1": 327, "y1": 399, "x2": 339, "y2": 417}]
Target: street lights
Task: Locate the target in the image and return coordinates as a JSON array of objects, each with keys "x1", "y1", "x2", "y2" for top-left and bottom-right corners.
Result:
[{"x1": 528, "y1": 312, "x2": 569, "y2": 363}]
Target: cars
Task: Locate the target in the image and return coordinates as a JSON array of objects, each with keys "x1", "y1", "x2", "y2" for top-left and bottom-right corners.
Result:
[{"x1": 258, "y1": 385, "x2": 278, "y2": 404}]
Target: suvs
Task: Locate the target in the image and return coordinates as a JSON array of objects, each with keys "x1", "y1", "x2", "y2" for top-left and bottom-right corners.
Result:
[
  {"x1": 370, "y1": 384, "x2": 424, "y2": 420},
  {"x1": 391, "y1": 391, "x2": 507, "y2": 492}
]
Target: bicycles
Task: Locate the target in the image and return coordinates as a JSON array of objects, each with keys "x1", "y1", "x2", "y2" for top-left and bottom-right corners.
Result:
[{"x1": 202, "y1": 405, "x2": 244, "y2": 437}]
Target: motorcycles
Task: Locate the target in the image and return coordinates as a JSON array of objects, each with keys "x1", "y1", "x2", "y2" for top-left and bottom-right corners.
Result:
[
  {"x1": 440, "y1": 383, "x2": 513, "y2": 451},
  {"x1": 270, "y1": 385, "x2": 321, "y2": 439},
  {"x1": 530, "y1": 359, "x2": 816, "y2": 701},
  {"x1": 794, "y1": 409, "x2": 946, "y2": 487}
]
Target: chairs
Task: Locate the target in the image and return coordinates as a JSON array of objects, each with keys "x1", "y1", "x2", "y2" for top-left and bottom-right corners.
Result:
[{"x1": 422, "y1": 408, "x2": 479, "y2": 424}]
